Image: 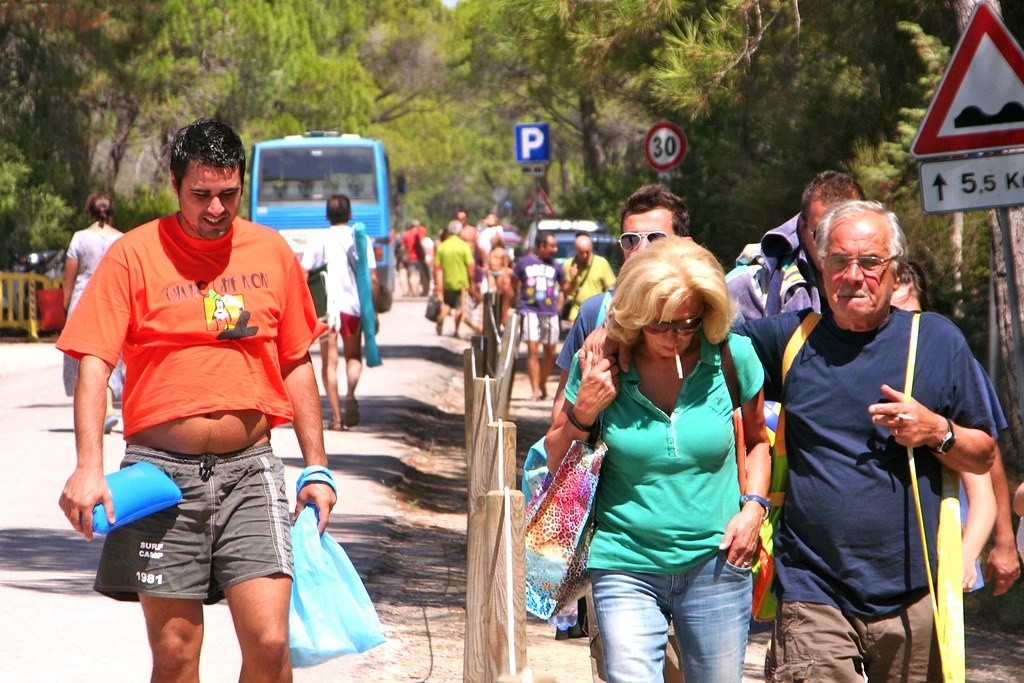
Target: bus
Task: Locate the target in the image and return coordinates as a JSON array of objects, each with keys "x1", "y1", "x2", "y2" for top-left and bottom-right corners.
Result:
[{"x1": 247, "y1": 131, "x2": 394, "y2": 313}]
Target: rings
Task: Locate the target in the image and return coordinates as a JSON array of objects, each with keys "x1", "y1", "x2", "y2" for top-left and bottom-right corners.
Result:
[
  {"x1": 743, "y1": 561, "x2": 749, "y2": 567},
  {"x1": 898, "y1": 414, "x2": 904, "y2": 427},
  {"x1": 895, "y1": 428, "x2": 899, "y2": 436}
]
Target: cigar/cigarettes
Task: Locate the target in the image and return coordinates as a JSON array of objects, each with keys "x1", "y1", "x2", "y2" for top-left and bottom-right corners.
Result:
[{"x1": 673, "y1": 347, "x2": 683, "y2": 379}]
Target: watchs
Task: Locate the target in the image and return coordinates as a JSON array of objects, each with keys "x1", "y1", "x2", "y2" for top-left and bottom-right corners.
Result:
[
  {"x1": 740, "y1": 495, "x2": 771, "y2": 520},
  {"x1": 926, "y1": 417, "x2": 956, "y2": 455}
]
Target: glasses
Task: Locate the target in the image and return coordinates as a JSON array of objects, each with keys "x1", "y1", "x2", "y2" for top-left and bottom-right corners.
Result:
[
  {"x1": 619, "y1": 231, "x2": 669, "y2": 251},
  {"x1": 823, "y1": 255, "x2": 901, "y2": 278},
  {"x1": 642, "y1": 314, "x2": 705, "y2": 335}
]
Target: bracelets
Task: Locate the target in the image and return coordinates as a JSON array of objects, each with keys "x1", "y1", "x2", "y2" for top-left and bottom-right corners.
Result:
[{"x1": 567, "y1": 406, "x2": 598, "y2": 433}]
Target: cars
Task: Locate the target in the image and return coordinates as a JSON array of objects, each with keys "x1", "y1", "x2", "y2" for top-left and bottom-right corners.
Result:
[{"x1": 518, "y1": 220, "x2": 627, "y2": 281}]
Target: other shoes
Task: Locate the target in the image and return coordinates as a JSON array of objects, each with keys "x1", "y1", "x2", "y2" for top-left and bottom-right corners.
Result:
[{"x1": 529, "y1": 388, "x2": 553, "y2": 401}]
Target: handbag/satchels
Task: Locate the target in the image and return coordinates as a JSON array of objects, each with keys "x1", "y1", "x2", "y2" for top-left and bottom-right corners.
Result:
[
  {"x1": 424, "y1": 294, "x2": 441, "y2": 321},
  {"x1": 282, "y1": 467, "x2": 386, "y2": 669},
  {"x1": 521, "y1": 343, "x2": 607, "y2": 622},
  {"x1": 718, "y1": 340, "x2": 748, "y2": 497}
]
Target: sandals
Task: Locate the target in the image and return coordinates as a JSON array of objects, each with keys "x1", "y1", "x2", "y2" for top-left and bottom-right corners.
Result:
[
  {"x1": 344, "y1": 397, "x2": 359, "y2": 428},
  {"x1": 328, "y1": 419, "x2": 344, "y2": 433}
]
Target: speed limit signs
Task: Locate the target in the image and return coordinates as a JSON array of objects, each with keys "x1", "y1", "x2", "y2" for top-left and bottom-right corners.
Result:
[{"x1": 644, "y1": 123, "x2": 688, "y2": 172}]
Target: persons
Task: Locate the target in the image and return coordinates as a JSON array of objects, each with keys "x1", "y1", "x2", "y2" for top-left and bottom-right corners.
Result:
[
  {"x1": 61, "y1": 192, "x2": 127, "y2": 433},
  {"x1": 396, "y1": 170, "x2": 1023, "y2": 683},
  {"x1": 578, "y1": 200, "x2": 998, "y2": 683},
  {"x1": 293, "y1": 194, "x2": 378, "y2": 429},
  {"x1": 59, "y1": 123, "x2": 336, "y2": 683},
  {"x1": 542, "y1": 236, "x2": 771, "y2": 683}
]
